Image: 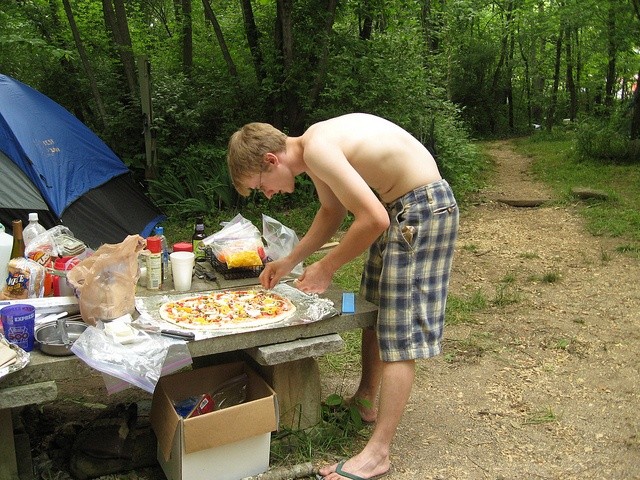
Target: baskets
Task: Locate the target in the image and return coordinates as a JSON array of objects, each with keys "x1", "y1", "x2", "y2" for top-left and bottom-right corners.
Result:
[{"x1": 205, "y1": 248, "x2": 274, "y2": 281}]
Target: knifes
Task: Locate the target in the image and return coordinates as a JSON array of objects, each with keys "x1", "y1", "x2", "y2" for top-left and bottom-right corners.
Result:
[{"x1": 126, "y1": 322, "x2": 195, "y2": 342}]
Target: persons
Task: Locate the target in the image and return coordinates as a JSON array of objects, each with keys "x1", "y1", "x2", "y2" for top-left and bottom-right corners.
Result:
[{"x1": 228, "y1": 112, "x2": 460, "y2": 480}]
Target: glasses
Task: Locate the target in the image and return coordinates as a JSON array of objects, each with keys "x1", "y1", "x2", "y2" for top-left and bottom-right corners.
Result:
[{"x1": 255, "y1": 159, "x2": 266, "y2": 194}]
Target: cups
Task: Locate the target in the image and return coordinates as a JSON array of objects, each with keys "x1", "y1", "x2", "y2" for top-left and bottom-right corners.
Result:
[
  {"x1": 0, "y1": 304, "x2": 36, "y2": 352},
  {"x1": 169, "y1": 251, "x2": 195, "y2": 291}
]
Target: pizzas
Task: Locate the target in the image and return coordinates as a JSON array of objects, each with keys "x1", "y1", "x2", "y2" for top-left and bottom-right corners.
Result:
[{"x1": 159, "y1": 291, "x2": 295, "y2": 329}]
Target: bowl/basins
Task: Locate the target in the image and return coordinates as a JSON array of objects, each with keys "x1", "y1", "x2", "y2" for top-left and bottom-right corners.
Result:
[{"x1": 34, "y1": 320, "x2": 95, "y2": 356}]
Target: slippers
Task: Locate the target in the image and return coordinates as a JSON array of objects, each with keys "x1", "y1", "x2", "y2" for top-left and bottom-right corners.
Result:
[
  {"x1": 321, "y1": 398, "x2": 376, "y2": 426},
  {"x1": 320, "y1": 457, "x2": 390, "y2": 479}
]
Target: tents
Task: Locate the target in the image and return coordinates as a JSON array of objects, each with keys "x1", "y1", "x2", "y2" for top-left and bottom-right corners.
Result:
[{"x1": 0, "y1": 70, "x2": 166, "y2": 251}]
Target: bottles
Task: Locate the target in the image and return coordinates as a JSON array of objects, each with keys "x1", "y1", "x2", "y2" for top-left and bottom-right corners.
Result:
[
  {"x1": 155, "y1": 227, "x2": 168, "y2": 280},
  {"x1": 10, "y1": 219, "x2": 25, "y2": 258},
  {"x1": 173, "y1": 242, "x2": 196, "y2": 276},
  {"x1": 22, "y1": 212, "x2": 47, "y2": 247},
  {"x1": 192, "y1": 224, "x2": 207, "y2": 262}
]
